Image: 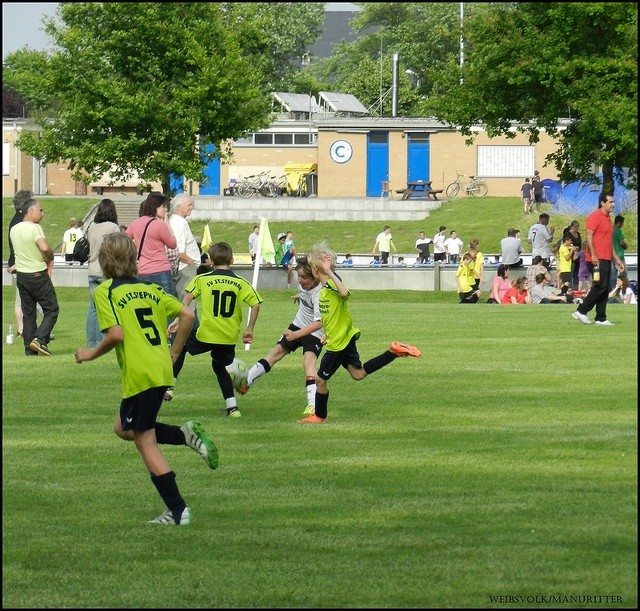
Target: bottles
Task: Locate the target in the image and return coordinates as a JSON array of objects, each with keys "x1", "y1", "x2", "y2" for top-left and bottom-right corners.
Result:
[
  {"x1": 5, "y1": 321, "x2": 15, "y2": 346},
  {"x1": 593, "y1": 265, "x2": 600, "y2": 283}
]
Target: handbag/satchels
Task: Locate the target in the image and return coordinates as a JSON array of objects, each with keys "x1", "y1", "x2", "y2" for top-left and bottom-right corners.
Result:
[{"x1": 73, "y1": 220, "x2": 93, "y2": 261}]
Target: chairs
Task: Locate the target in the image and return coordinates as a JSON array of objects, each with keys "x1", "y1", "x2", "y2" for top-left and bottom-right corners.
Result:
[{"x1": 381, "y1": 181, "x2": 393, "y2": 200}]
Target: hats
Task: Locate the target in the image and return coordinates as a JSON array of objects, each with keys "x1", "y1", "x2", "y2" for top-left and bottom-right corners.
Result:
[{"x1": 277, "y1": 232, "x2": 286, "y2": 240}]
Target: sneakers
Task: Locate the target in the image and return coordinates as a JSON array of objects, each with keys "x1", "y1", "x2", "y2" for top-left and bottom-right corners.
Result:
[
  {"x1": 181, "y1": 420, "x2": 220, "y2": 471},
  {"x1": 302, "y1": 405, "x2": 315, "y2": 415},
  {"x1": 164, "y1": 386, "x2": 174, "y2": 400},
  {"x1": 296, "y1": 413, "x2": 327, "y2": 423},
  {"x1": 30, "y1": 337, "x2": 53, "y2": 355},
  {"x1": 235, "y1": 382, "x2": 249, "y2": 394},
  {"x1": 595, "y1": 319, "x2": 615, "y2": 326},
  {"x1": 389, "y1": 341, "x2": 422, "y2": 357},
  {"x1": 147, "y1": 506, "x2": 190, "y2": 526},
  {"x1": 572, "y1": 310, "x2": 592, "y2": 323},
  {"x1": 226, "y1": 408, "x2": 242, "y2": 419}
]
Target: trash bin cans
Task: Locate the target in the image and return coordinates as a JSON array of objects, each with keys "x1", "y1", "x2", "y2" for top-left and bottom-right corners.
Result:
[{"x1": 285, "y1": 164, "x2": 317, "y2": 194}]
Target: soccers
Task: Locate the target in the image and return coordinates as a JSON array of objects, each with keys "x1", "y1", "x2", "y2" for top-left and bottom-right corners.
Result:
[{"x1": 225, "y1": 358, "x2": 248, "y2": 388}]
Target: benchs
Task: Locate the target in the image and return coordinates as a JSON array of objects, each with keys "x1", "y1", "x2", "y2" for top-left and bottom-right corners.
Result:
[
  {"x1": 396, "y1": 188, "x2": 443, "y2": 200},
  {"x1": 91, "y1": 183, "x2": 152, "y2": 196}
]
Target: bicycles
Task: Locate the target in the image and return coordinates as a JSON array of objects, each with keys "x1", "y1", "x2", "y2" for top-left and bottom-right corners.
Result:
[
  {"x1": 446, "y1": 170, "x2": 488, "y2": 196},
  {"x1": 233, "y1": 172, "x2": 265, "y2": 197},
  {"x1": 296, "y1": 172, "x2": 306, "y2": 197},
  {"x1": 238, "y1": 170, "x2": 276, "y2": 198},
  {"x1": 262, "y1": 174, "x2": 291, "y2": 197}
]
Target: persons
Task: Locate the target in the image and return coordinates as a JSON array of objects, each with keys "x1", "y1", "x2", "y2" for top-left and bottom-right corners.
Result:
[
  {"x1": 516, "y1": 229, "x2": 521, "y2": 239},
  {"x1": 84, "y1": 198, "x2": 122, "y2": 349},
  {"x1": 520, "y1": 178, "x2": 535, "y2": 214},
  {"x1": 196, "y1": 241, "x2": 212, "y2": 274},
  {"x1": 166, "y1": 193, "x2": 201, "y2": 310},
  {"x1": 372, "y1": 226, "x2": 397, "y2": 264},
  {"x1": 485, "y1": 263, "x2": 513, "y2": 304},
  {"x1": 483, "y1": 256, "x2": 489, "y2": 263},
  {"x1": 6, "y1": 189, "x2": 59, "y2": 341},
  {"x1": 500, "y1": 277, "x2": 531, "y2": 304},
  {"x1": 442, "y1": 230, "x2": 464, "y2": 263},
  {"x1": 229, "y1": 255, "x2": 328, "y2": 415},
  {"x1": 73, "y1": 231, "x2": 221, "y2": 527},
  {"x1": 341, "y1": 253, "x2": 354, "y2": 268},
  {"x1": 460, "y1": 237, "x2": 486, "y2": 286},
  {"x1": 273, "y1": 232, "x2": 299, "y2": 289},
  {"x1": 60, "y1": 216, "x2": 85, "y2": 266},
  {"x1": 500, "y1": 227, "x2": 524, "y2": 267},
  {"x1": 573, "y1": 240, "x2": 591, "y2": 293},
  {"x1": 284, "y1": 231, "x2": 295, "y2": 264},
  {"x1": 397, "y1": 256, "x2": 407, "y2": 265},
  {"x1": 525, "y1": 254, "x2": 552, "y2": 293},
  {"x1": 529, "y1": 170, "x2": 541, "y2": 213},
  {"x1": 610, "y1": 214, "x2": 629, "y2": 292},
  {"x1": 433, "y1": 225, "x2": 447, "y2": 264},
  {"x1": 562, "y1": 220, "x2": 582, "y2": 292},
  {"x1": 414, "y1": 231, "x2": 434, "y2": 263},
  {"x1": 467, "y1": 248, "x2": 486, "y2": 303},
  {"x1": 607, "y1": 275, "x2": 637, "y2": 305},
  {"x1": 247, "y1": 225, "x2": 259, "y2": 264},
  {"x1": 527, "y1": 213, "x2": 555, "y2": 271},
  {"x1": 123, "y1": 190, "x2": 177, "y2": 345},
  {"x1": 412, "y1": 256, "x2": 423, "y2": 264},
  {"x1": 558, "y1": 235, "x2": 577, "y2": 295},
  {"x1": 492, "y1": 255, "x2": 503, "y2": 264},
  {"x1": 549, "y1": 243, "x2": 560, "y2": 290},
  {"x1": 369, "y1": 255, "x2": 385, "y2": 268},
  {"x1": 532, "y1": 175, "x2": 544, "y2": 212},
  {"x1": 296, "y1": 243, "x2": 422, "y2": 424},
  {"x1": 455, "y1": 253, "x2": 480, "y2": 303},
  {"x1": 9, "y1": 198, "x2": 60, "y2": 358},
  {"x1": 159, "y1": 240, "x2": 266, "y2": 419},
  {"x1": 531, "y1": 272, "x2": 575, "y2": 304},
  {"x1": 424, "y1": 255, "x2": 433, "y2": 264},
  {"x1": 571, "y1": 190, "x2": 626, "y2": 327},
  {"x1": 76, "y1": 220, "x2": 85, "y2": 238}
]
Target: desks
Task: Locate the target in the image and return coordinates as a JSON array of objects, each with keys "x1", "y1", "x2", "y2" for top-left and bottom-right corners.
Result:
[{"x1": 400, "y1": 182, "x2": 438, "y2": 201}]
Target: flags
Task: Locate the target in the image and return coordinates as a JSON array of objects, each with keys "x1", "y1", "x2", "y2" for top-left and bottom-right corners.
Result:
[
  {"x1": 258, "y1": 217, "x2": 276, "y2": 263},
  {"x1": 200, "y1": 223, "x2": 213, "y2": 253}
]
